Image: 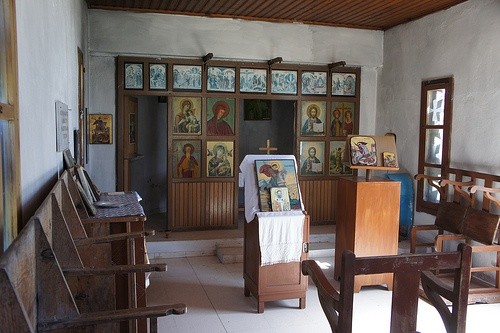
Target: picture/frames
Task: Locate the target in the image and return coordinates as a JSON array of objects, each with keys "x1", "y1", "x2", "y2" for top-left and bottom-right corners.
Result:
[
  {"x1": 331, "y1": 72, "x2": 358, "y2": 97},
  {"x1": 270, "y1": 69, "x2": 299, "y2": 96},
  {"x1": 301, "y1": 70, "x2": 328, "y2": 96},
  {"x1": 206, "y1": 65, "x2": 236, "y2": 93},
  {"x1": 171, "y1": 63, "x2": 203, "y2": 92},
  {"x1": 239, "y1": 67, "x2": 268, "y2": 94},
  {"x1": 124, "y1": 61, "x2": 145, "y2": 90},
  {"x1": 88, "y1": 114, "x2": 114, "y2": 145},
  {"x1": 148, "y1": 62, "x2": 169, "y2": 92}
]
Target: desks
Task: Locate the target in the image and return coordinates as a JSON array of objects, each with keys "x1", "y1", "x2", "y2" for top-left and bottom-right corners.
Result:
[{"x1": 95, "y1": 191, "x2": 150, "y2": 333}]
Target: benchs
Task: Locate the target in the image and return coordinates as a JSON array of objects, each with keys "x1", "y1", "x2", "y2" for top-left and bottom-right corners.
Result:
[
  {"x1": 410, "y1": 167, "x2": 500, "y2": 294},
  {"x1": 0, "y1": 169, "x2": 188, "y2": 333}
]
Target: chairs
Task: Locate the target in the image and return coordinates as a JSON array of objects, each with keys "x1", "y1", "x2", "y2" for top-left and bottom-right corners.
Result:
[{"x1": 301, "y1": 243, "x2": 473, "y2": 333}]
